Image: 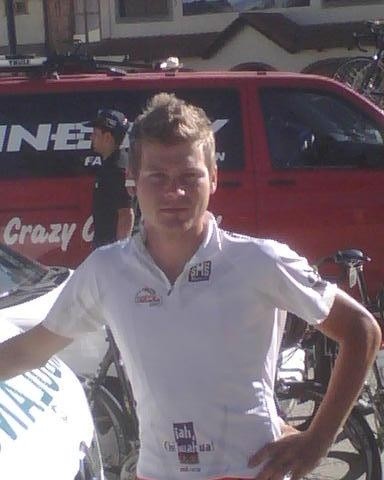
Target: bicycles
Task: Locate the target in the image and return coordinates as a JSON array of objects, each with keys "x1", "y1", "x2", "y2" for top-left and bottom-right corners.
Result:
[
  {"x1": 73, "y1": 321, "x2": 142, "y2": 480},
  {"x1": 274, "y1": 246, "x2": 384, "y2": 480},
  {"x1": 334, "y1": 18, "x2": 384, "y2": 111}
]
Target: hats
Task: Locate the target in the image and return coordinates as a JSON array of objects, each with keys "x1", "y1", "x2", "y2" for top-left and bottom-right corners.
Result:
[{"x1": 82, "y1": 109, "x2": 130, "y2": 133}]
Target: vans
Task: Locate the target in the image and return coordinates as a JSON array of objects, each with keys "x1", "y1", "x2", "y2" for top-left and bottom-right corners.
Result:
[{"x1": 0, "y1": 67, "x2": 384, "y2": 332}]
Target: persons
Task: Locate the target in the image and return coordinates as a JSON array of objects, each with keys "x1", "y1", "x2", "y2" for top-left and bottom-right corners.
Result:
[
  {"x1": 0, "y1": 93, "x2": 382, "y2": 480},
  {"x1": 83, "y1": 110, "x2": 142, "y2": 249}
]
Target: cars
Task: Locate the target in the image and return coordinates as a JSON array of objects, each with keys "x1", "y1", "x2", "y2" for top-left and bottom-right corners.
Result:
[{"x1": 0, "y1": 240, "x2": 129, "y2": 417}]
